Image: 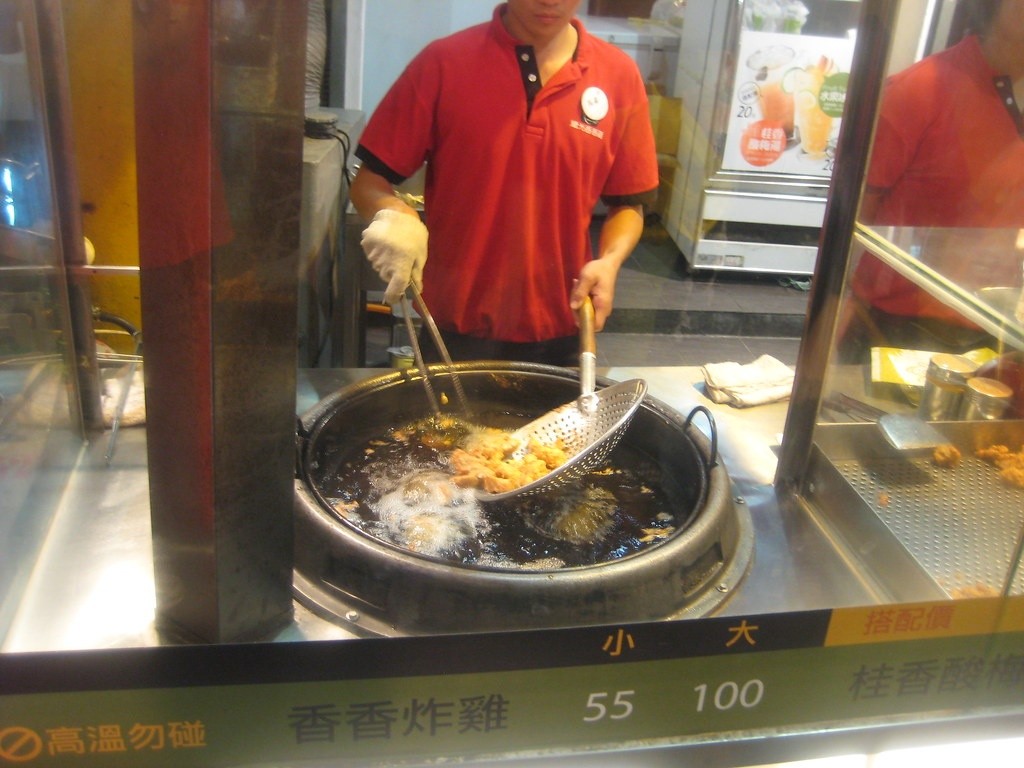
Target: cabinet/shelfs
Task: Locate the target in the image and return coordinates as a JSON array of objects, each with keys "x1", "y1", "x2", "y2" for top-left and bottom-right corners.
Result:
[{"x1": 776, "y1": 0, "x2": 1024, "y2": 602}]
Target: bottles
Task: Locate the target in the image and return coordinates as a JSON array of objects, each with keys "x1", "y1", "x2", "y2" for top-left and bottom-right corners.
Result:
[
  {"x1": 919, "y1": 352, "x2": 979, "y2": 421},
  {"x1": 957, "y1": 377, "x2": 1014, "y2": 421}
]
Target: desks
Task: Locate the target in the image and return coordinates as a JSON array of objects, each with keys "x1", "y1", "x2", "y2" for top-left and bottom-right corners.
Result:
[{"x1": 330, "y1": 163, "x2": 425, "y2": 368}]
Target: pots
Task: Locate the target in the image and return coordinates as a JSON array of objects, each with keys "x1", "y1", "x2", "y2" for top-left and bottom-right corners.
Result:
[{"x1": 284, "y1": 359, "x2": 743, "y2": 621}]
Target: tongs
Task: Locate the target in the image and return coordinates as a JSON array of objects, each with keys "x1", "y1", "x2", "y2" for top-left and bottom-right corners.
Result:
[{"x1": 400, "y1": 278, "x2": 473, "y2": 428}]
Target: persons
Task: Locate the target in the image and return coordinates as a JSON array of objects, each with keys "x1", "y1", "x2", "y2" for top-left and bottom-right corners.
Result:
[
  {"x1": 346, "y1": 1, "x2": 661, "y2": 371},
  {"x1": 831, "y1": 0, "x2": 1024, "y2": 366}
]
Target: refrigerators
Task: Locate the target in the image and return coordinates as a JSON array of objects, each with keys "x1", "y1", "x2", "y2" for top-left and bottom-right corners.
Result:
[{"x1": 659, "y1": 0, "x2": 957, "y2": 276}]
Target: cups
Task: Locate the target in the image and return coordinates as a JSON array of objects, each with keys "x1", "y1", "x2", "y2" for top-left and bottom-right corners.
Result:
[{"x1": 743, "y1": 45, "x2": 837, "y2": 157}]
